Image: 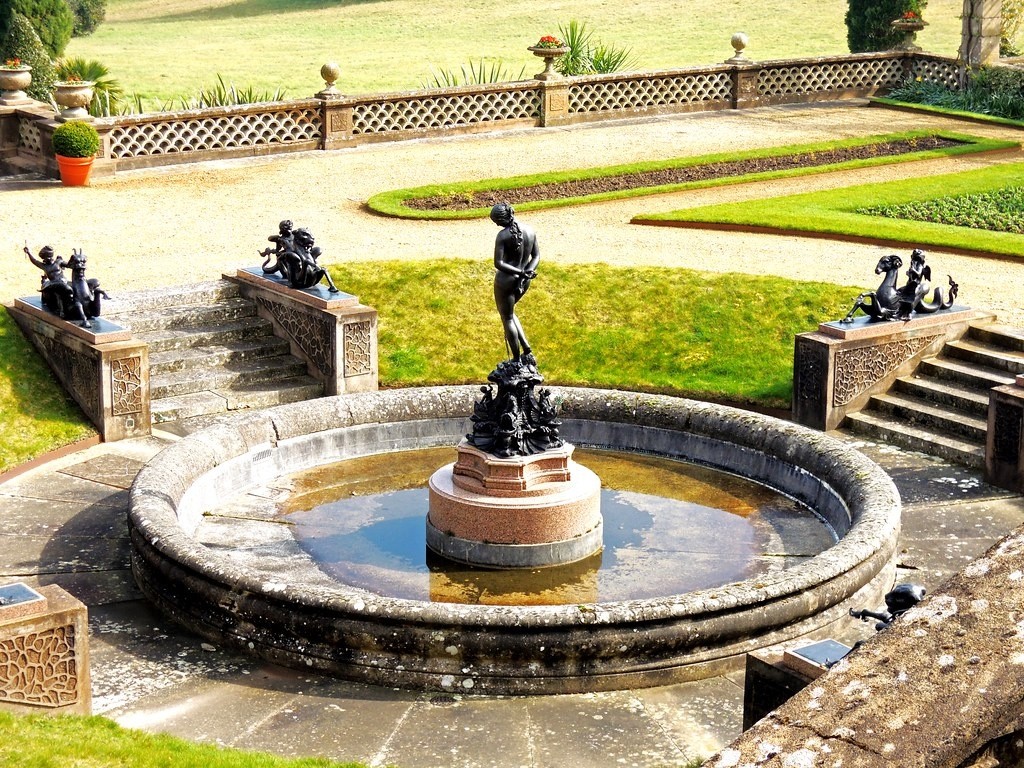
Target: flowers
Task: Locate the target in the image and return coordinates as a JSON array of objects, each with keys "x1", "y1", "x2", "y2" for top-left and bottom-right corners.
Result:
[
  {"x1": 5, "y1": 57, "x2": 22, "y2": 69},
  {"x1": 67, "y1": 77, "x2": 81, "y2": 85},
  {"x1": 899, "y1": 11, "x2": 921, "y2": 23},
  {"x1": 535, "y1": 36, "x2": 566, "y2": 50}
]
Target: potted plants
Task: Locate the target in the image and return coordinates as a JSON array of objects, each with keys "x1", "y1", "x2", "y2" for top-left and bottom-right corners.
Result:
[
  {"x1": 51, "y1": 119, "x2": 99, "y2": 185},
  {"x1": 0, "y1": 65, "x2": 33, "y2": 106}
]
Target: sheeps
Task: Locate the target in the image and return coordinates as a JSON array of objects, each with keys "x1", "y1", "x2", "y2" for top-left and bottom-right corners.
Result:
[{"x1": 840, "y1": 255, "x2": 930, "y2": 323}]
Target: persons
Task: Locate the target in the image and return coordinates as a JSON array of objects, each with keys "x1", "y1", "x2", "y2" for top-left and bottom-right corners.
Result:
[
  {"x1": 490, "y1": 204, "x2": 541, "y2": 363},
  {"x1": 23, "y1": 245, "x2": 73, "y2": 299},
  {"x1": 895, "y1": 249, "x2": 925, "y2": 320},
  {"x1": 268, "y1": 219, "x2": 301, "y2": 253}
]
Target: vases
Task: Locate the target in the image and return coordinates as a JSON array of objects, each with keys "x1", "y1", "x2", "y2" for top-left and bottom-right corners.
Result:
[
  {"x1": 53, "y1": 80, "x2": 95, "y2": 122},
  {"x1": 891, "y1": 19, "x2": 929, "y2": 51},
  {"x1": 527, "y1": 45, "x2": 571, "y2": 80}
]
